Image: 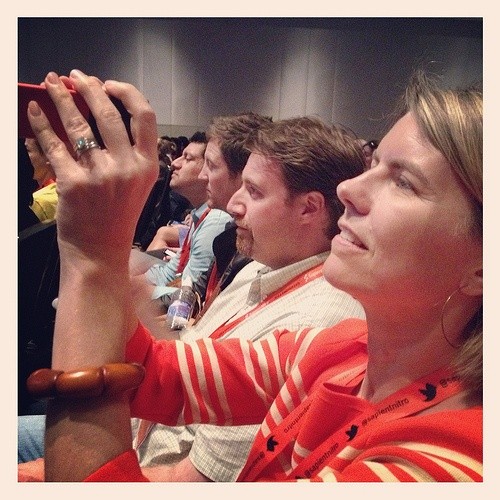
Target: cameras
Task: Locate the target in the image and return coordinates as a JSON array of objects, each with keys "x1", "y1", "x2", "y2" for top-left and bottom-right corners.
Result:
[{"x1": 18, "y1": 82, "x2": 134, "y2": 148}]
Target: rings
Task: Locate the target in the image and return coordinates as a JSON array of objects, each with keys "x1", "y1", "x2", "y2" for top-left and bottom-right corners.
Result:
[{"x1": 74, "y1": 137, "x2": 100, "y2": 158}]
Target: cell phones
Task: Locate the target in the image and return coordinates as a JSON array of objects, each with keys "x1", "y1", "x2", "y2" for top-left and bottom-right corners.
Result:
[{"x1": 162, "y1": 248, "x2": 176, "y2": 256}]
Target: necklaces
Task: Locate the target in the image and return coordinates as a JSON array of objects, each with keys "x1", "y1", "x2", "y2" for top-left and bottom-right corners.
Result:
[
  {"x1": 175, "y1": 206, "x2": 211, "y2": 274},
  {"x1": 209, "y1": 259, "x2": 324, "y2": 340},
  {"x1": 234, "y1": 356, "x2": 469, "y2": 483},
  {"x1": 36, "y1": 176, "x2": 56, "y2": 193},
  {"x1": 192, "y1": 250, "x2": 239, "y2": 326}
]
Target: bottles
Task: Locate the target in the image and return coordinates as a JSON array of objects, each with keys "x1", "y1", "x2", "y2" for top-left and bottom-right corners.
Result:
[{"x1": 164, "y1": 280, "x2": 196, "y2": 332}]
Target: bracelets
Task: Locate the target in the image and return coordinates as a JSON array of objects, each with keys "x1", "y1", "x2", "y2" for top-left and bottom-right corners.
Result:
[{"x1": 28, "y1": 362, "x2": 146, "y2": 399}]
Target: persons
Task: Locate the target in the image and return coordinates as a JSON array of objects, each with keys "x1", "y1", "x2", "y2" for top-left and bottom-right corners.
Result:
[
  {"x1": 20, "y1": 113, "x2": 277, "y2": 339},
  {"x1": 28, "y1": 53, "x2": 483, "y2": 482},
  {"x1": 18, "y1": 117, "x2": 369, "y2": 482}
]
